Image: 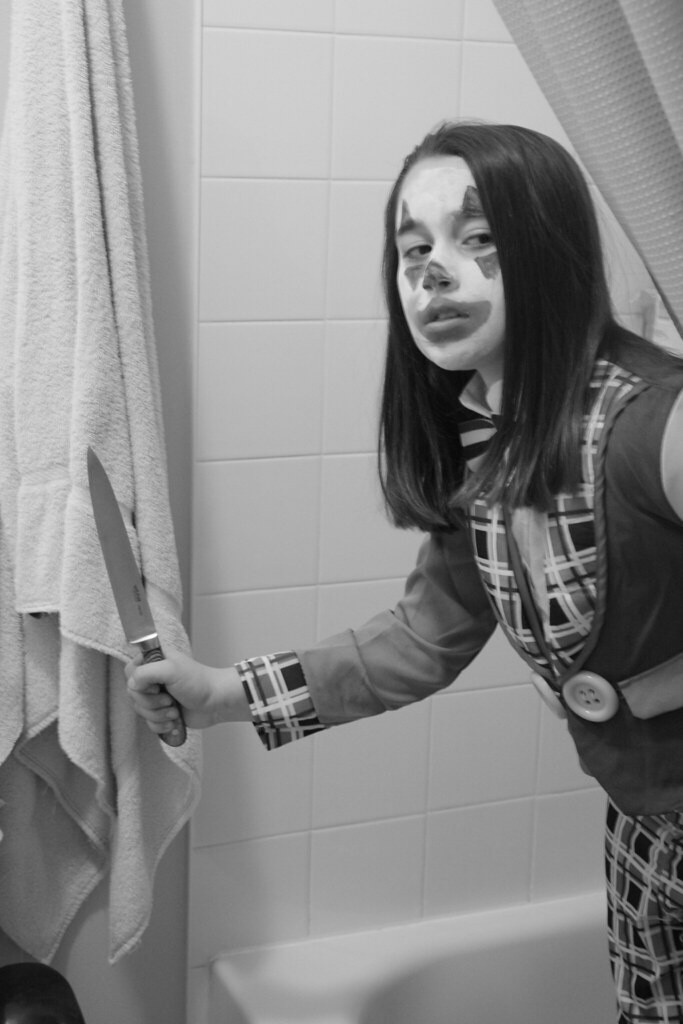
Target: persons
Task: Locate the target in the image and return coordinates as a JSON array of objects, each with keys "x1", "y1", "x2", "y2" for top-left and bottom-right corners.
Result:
[{"x1": 125, "y1": 122, "x2": 683, "y2": 1023}]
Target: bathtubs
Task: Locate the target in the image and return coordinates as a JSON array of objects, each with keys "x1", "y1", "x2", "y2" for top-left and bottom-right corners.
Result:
[{"x1": 211, "y1": 881, "x2": 617, "y2": 1023}]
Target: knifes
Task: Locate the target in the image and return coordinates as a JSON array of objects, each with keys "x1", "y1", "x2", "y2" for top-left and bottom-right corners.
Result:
[{"x1": 85, "y1": 444, "x2": 187, "y2": 748}]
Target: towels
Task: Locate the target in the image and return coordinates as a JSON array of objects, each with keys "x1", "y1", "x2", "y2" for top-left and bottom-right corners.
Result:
[{"x1": 0, "y1": 0, "x2": 204, "y2": 973}]
